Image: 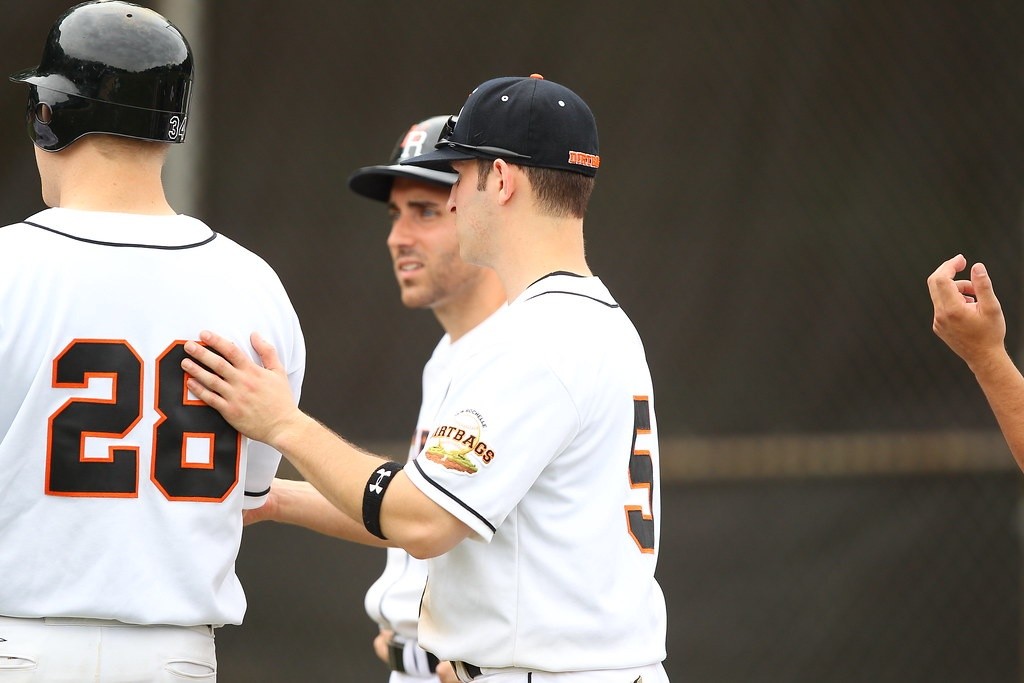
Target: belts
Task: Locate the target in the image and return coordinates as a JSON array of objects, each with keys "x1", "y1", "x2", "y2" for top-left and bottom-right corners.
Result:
[{"x1": 387, "y1": 644, "x2": 483, "y2": 682}]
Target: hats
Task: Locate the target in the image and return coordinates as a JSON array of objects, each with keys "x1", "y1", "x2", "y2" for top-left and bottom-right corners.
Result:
[{"x1": 400, "y1": 73, "x2": 599, "y2": 180}]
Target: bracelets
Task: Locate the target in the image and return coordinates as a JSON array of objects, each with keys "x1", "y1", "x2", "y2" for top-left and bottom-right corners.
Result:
[{"x1": 361, "y1": 462, "x2": 404, "y2": 542}]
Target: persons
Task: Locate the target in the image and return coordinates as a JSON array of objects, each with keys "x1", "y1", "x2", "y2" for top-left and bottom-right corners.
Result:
[
  {"x1": 927, "y1": 254, "x2": 1024, "y2": 482},
  {"x1": 0, "y1": 1, "x2": 307, "y2": 683},
  {"x1": 183, "y1": 73, "x2": 671, "y2": 683}
]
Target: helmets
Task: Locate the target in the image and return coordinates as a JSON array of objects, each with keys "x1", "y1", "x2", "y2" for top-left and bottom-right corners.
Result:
[
  {"x1": 347, "y1": 116, "x2": 464, "y2": 203},
  {"x1": 9, "y1": 2, "x2": 194, "y2": 152}
]
